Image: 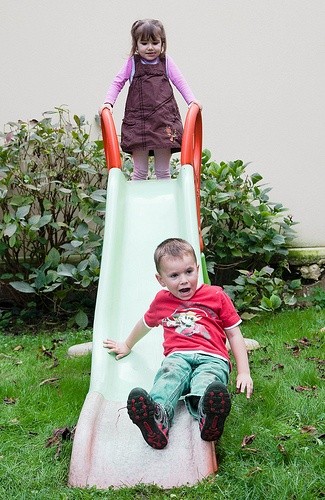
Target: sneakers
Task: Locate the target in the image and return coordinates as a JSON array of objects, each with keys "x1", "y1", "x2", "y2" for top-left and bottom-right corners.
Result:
[
  {"x1": 197, "y1": 381, "x2": 232, "y2": 441},
  {"x1": 127, "y1": 387, "x2": 170, "y2": 449}
]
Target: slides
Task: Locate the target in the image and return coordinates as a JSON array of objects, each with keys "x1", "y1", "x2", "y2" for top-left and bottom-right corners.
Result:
[{"x1": 67, "y1": 164, "x2": 218, "y2": 489}]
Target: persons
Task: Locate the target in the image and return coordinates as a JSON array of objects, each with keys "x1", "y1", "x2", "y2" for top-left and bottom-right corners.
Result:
[
  {"x1": 98, "y1": 19, "x2": 202, "y2": 179},
  {"x1": 103, "y1": 238, "x2": 254, "y2": 450}
]
